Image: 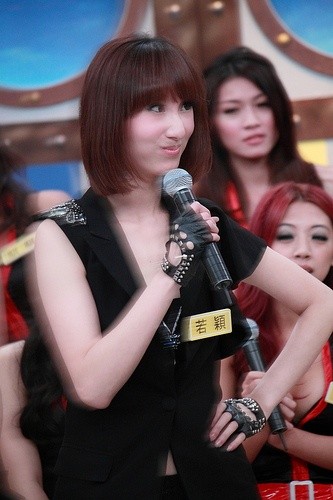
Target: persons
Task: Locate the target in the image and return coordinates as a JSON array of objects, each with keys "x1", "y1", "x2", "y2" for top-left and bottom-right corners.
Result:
[
  {"x1": 188, "y1": 46, "x2": 326, "y2": 282},
  {"x1": 6, "y1": 33, "x2": 333, "y2": 500},
  {"x1": 216, "y1": 182, "x2": 333, "y2": 500},
  {"x1": 0, "y1": 188, "x2": 76, "y2": 500}
]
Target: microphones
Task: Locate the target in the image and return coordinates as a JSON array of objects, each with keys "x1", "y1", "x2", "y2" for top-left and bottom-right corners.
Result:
[
  {"x1": 162, "y1": 168, "x2": 235, "y2": 305},
  {"x1": 242, "y1": 317, "x2": 289, "y2": 452}
]
